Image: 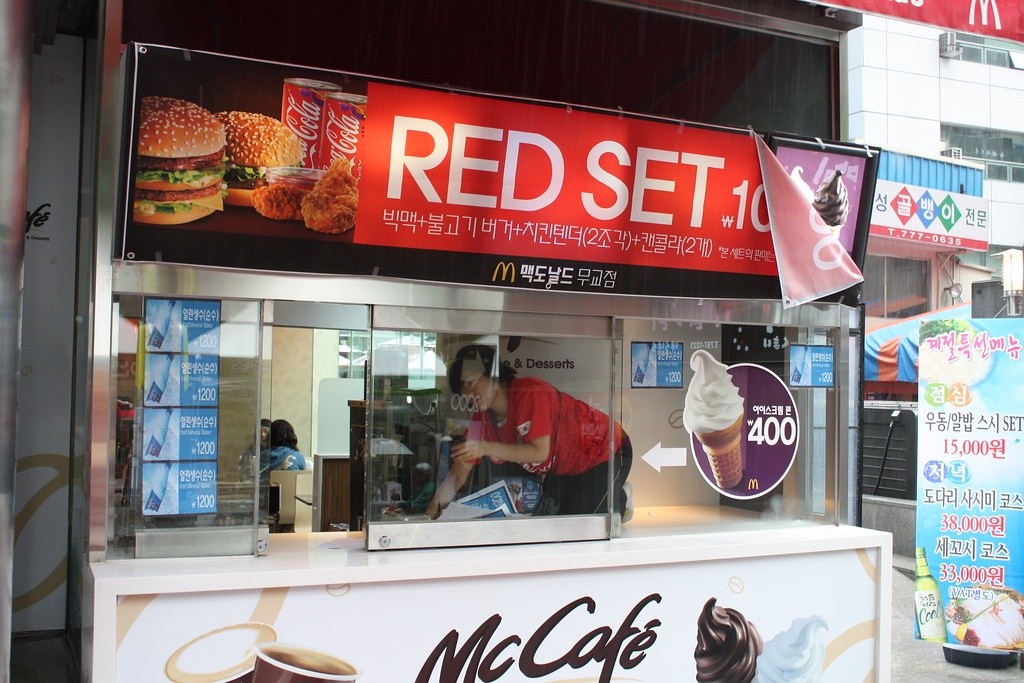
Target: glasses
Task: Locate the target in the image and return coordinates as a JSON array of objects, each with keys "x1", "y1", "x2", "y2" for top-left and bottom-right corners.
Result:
[{"x1": 465, "y1": 373, "x2": 482, "y2": 392}]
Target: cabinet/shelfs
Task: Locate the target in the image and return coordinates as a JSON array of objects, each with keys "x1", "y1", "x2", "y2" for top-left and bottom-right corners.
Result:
[{"x1": 345, "y1": 398, "x2": 435, "y2": 532}]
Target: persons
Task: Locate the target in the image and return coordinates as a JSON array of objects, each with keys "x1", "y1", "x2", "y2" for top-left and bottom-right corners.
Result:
[
  {"x1": 411, "y1": 343, "x2": 635, "y2": 524},
  {"x1": 115, "y1": 395, "x2": 135, "y2": 479},
  {"x1": 368, "y1": 474, "x2": 387, "y2": 521},
  {"x1": 386, "y1": 461, "x2": 436, "y2": 515},
  {"x1": 238, "y1": 418, "x2": 307, "y2": 516},
  {"x1": 391, "y1": 488, "x2": 401, "y2": 501}
]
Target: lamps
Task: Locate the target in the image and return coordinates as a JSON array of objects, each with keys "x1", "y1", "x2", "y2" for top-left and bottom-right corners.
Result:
[{"x1": 944, "y1": 283, "x2": 961, "y2": 298}]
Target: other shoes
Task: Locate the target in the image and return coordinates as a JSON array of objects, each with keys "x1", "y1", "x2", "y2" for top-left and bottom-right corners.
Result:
[{"x1": 620, "y1": 481, "x2": 634, "y2": 524}]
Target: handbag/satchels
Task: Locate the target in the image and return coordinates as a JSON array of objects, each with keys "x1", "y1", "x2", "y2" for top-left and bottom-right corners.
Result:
[{"x1": 531, "y1": 468, "x2": 561, "y2": 516}]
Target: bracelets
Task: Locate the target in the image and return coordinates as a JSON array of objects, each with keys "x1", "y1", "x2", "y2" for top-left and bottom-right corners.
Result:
[{"x1": 423, "y1": 514, "x2": 431, "y2": 521}]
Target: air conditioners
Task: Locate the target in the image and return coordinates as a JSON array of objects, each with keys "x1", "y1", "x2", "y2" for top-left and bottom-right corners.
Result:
[
  {"x1": 939, "y1": 33, "x2": 963, "y2": 57},
  {"x1": 942, "y1": 149, "x2": 963, "y2": 160}
]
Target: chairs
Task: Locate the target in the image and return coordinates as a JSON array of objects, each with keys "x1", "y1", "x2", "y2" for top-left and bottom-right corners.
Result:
[{"x1": 270, "y1": 471, "x2": 313, "y2": 533}]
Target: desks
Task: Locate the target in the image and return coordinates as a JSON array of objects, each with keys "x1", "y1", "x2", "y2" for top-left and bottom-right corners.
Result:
[{"x1": 295, "y1": 495, "x2": 313, "y2": 505}]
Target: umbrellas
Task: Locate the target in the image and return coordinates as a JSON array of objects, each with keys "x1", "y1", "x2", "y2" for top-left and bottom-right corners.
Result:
[
  {"x1": 338, "y1": 334, "x2": 448, "y2": 379},
  {"x1": 370, "y1": 434, "x2": 414, "y2": 482}
]
